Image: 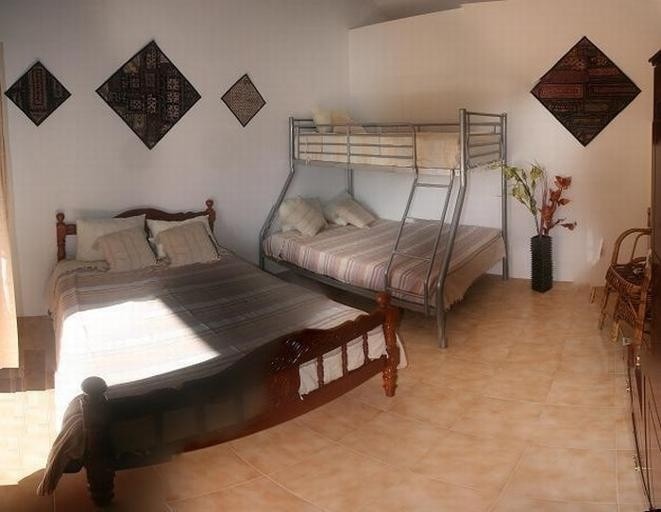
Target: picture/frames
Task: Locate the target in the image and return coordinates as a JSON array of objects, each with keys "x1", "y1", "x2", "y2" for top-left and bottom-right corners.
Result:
[
  {"x1": 94, "y1": 39, "x2": 201, "y2": 150},
  {"x1": 220, "y1": 73, "x2": 267, "y2": 128},
  {"x1": 528, "y1": 35, "x2": 643, "y2": 147},
  {"x1": 3, "y1": 60, "x2": 72, "y2": 127}
]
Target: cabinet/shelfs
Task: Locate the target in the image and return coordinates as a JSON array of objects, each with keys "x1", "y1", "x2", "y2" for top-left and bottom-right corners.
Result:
[{"x1": 627, "y1": 48, "x2": 661, "y2": 511}]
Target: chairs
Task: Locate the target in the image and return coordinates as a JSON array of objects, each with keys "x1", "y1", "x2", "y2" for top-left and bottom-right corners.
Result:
[{"x1": 599, "y1": 227, "x2": 651, "y2": 341}]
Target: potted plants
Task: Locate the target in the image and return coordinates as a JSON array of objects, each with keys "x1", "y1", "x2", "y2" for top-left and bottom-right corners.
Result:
[{"x1": 489, "y1": 159, "x2": 578, "y2": 293}]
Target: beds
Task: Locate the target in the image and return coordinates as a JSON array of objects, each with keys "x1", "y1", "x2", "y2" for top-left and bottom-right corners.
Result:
[
  {"x1": 36, "y1": 199, "x2": 408, "y2": 512},
  {"x1": 259, "y1": 109, "x2": 508, "y2": 349}
]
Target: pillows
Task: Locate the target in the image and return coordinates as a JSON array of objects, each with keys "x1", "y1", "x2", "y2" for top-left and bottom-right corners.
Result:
[
  {"x1": 75, "y1": 213, "x2": 146, "y2": 262},
  {"x1": 156, "y1": 222, "x2": 219, "y2": 266},
  {"x1": 331, "y1": 111, "x2": 366, "y2": 134},
  {"x1": 147, "y1": 214, "x2": 219, "y2": 260},
  {"x1": 311, "y1": 109, "x2": 353, "y2": 135},
  {"x1": 95, "y1": 225, "x2": 159, "y2": 273},
  {"x1": 279, "y1": 191, "x2": 375, "y2": 237}
]
